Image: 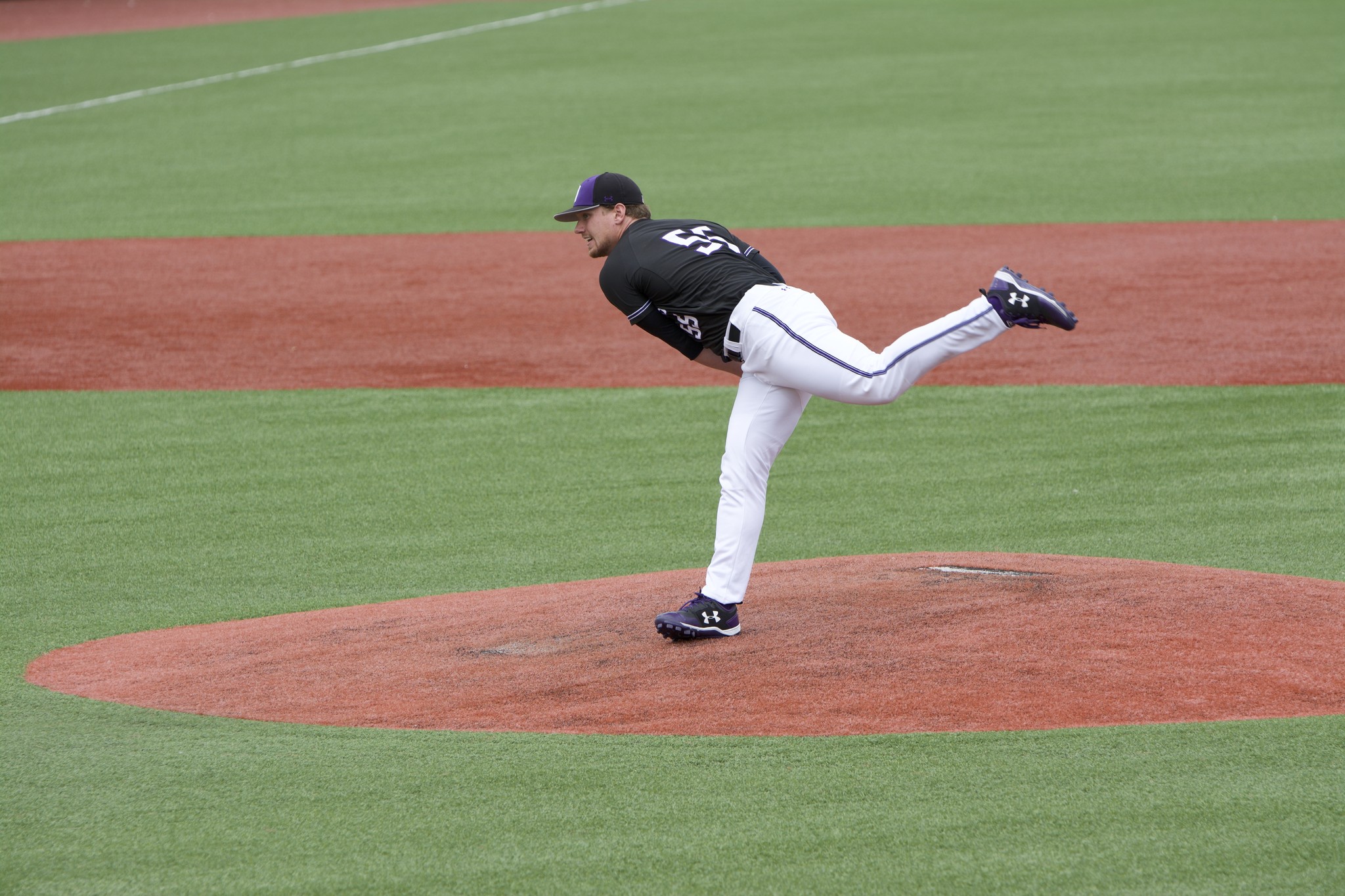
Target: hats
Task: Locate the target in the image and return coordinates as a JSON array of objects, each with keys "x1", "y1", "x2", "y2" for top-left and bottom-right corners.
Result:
[{"x1": 554, "y1": 173, "x2": 643, "y2": 222}]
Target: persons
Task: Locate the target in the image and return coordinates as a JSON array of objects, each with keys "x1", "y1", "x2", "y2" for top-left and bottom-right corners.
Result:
[{"x1": 554, "y1": 173, "x2": 1080, "y2": 642}]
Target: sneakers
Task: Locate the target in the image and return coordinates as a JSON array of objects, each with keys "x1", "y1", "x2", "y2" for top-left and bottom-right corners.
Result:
[
  {"x1": 979, "y1": 265, "x2": 1078, "y2": 332},
  {"x1": 654, "y1": 586, "x2": 740, "y2": 641}
]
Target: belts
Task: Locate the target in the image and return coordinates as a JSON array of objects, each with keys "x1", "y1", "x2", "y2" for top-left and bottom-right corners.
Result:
[{"x1": 727, "y1": 282, "x2": 779, "y2": 362}]
